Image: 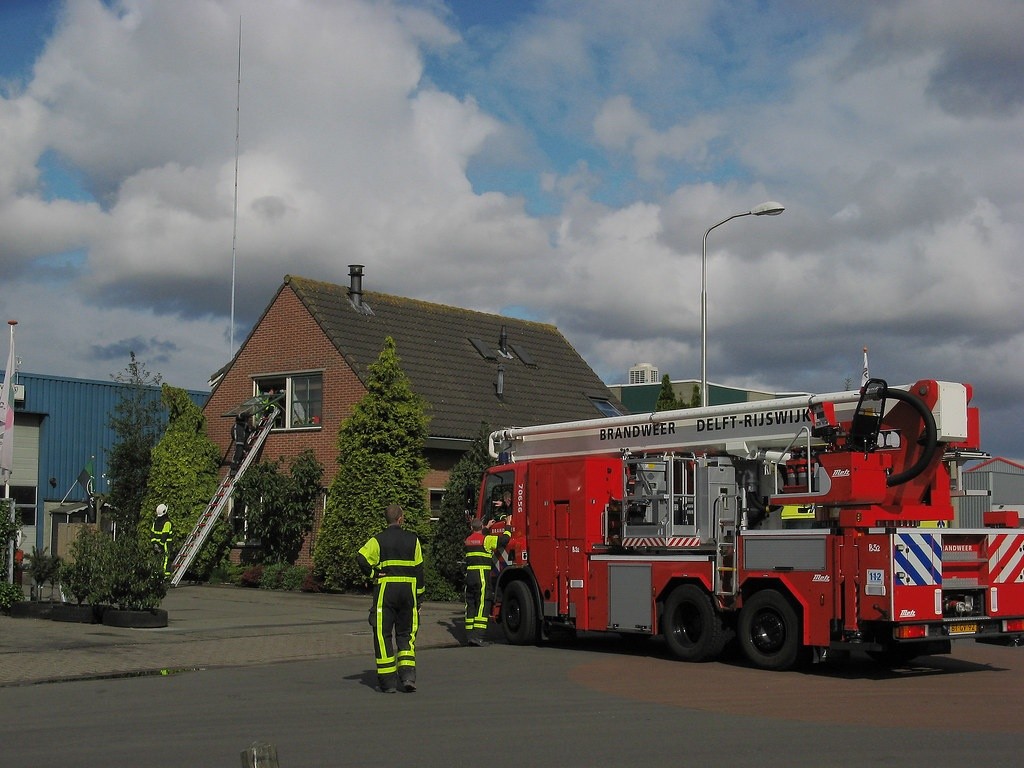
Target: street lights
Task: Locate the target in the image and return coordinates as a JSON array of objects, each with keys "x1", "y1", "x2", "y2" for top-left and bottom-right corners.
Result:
[{"x1": 699, "y1": 201, "x2": 786, "y2": 409}]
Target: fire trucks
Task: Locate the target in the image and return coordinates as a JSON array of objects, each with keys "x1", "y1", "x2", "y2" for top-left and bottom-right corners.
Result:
[{"x1": 463, "y1": 347, "x2": 1024, "y2": 671}]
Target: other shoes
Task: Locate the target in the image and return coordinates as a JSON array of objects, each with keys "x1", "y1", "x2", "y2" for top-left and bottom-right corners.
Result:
[
  {"x1": 230, "y1": 470, "x2": 237, "y2": 476},
  {"x1": 402, "y1": 680, "x2": 416, "y2": 692},
  {"x1": 468, "y1": 638, "x2": 489, "y2": 647},
  {"x1": 375, "y1": 685, "x2": 396, "y2": 692}
]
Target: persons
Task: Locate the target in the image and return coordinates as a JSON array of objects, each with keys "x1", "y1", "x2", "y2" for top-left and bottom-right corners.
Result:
[
  {"x1": 150, "y1": 504, "x2": 174, "y2": 580},
  {"x1": 230, "y1": 385, "x2": 286, "y2": 478},
  {"x1": 492, "y1": 490, "x2": 512, "y2": 523},
  {"x1": 464, "y1": 514, "x2": 512, "y2": 646},
  {"x1": 358, "y1": 504, "x2": 425, "y2": 693}
]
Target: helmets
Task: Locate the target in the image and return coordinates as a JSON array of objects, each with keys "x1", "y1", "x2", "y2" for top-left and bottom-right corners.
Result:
[{"x1": 156, "y1": 504, "x2": 168, "y2": 517}]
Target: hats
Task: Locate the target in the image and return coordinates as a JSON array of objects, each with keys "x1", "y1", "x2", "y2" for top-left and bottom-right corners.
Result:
[{"x1": 471, "y1": 518, "x2": 483, "y2": 528}]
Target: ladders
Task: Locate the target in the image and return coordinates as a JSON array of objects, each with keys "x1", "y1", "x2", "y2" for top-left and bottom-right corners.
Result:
[{"x1": 167, "y1": 405, "x2": 281, "y2": 589}]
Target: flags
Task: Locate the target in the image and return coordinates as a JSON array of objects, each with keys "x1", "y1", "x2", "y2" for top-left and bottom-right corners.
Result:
[
  {"x1": 0, "y1": 338, "x2": 17, "y2": 480},
  {"x1": 78, "y1": 457, "x2": 96, "y2": 521}
]
